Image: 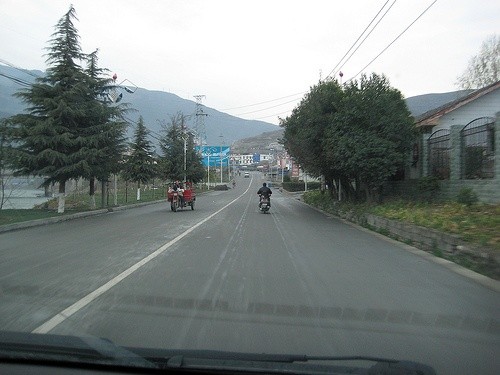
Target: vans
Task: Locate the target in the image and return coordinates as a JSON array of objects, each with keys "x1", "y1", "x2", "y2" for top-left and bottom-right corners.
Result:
[{"x1": 244, "y1": 171, "x2": 250, "y2": 179}]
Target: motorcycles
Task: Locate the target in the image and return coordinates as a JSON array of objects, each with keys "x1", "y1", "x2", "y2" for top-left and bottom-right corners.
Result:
[
  {"x1": 257, "y1": 192, "x2": 271, "y2": 214},
  {"x1": 168, "y1": 187, "x2": 197, "y2": 212}
]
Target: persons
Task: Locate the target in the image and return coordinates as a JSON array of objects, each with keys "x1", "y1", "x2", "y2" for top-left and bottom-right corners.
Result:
[
  {"x1": 172, "y1": 180, "x2": 184, "y2": 207},
  {"x1": 257, "y1": 183, "x2": 272, "y2": 207}
]
